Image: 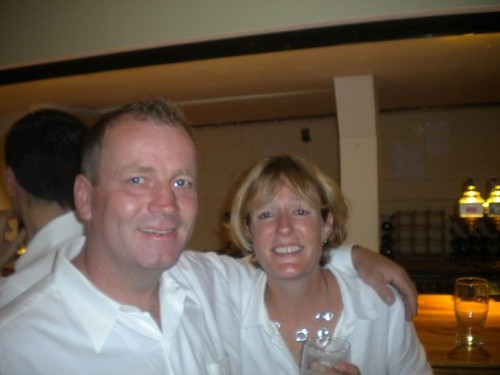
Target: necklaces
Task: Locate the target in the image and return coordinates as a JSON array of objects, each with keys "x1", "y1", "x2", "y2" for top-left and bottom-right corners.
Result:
[{"x1": 264, "y1": 269, "x2": 334, "y2": 349}]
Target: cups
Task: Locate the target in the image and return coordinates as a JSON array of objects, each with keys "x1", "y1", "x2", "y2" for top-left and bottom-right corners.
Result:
[
  {"x1": 300, "y1": 337, "x2": 352, "y2": 375},
  {"x1": 454, "y1": 277, "x2": 489, "y2": 352}
]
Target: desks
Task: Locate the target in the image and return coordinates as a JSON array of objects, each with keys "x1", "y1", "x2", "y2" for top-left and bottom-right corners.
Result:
[{"x1": 412, "y1": 293, "x2": 500, "y2": 375}]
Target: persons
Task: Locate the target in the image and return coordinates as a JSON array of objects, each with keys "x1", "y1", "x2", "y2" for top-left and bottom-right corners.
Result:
[
  {"x1": 222, "y1": 152, "x2": 433, "y2": 375},
  {"x1": 0, "y1": 95, "x2": 419, "y2": 375},
  {"x1": 0, "y1": 108, "x2": 90, "y2": 310}
]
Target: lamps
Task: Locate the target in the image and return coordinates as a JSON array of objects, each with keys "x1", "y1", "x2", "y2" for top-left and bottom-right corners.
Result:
[{"x1": 458, "y1": 186, "x2": 500, "y2": 231}]
